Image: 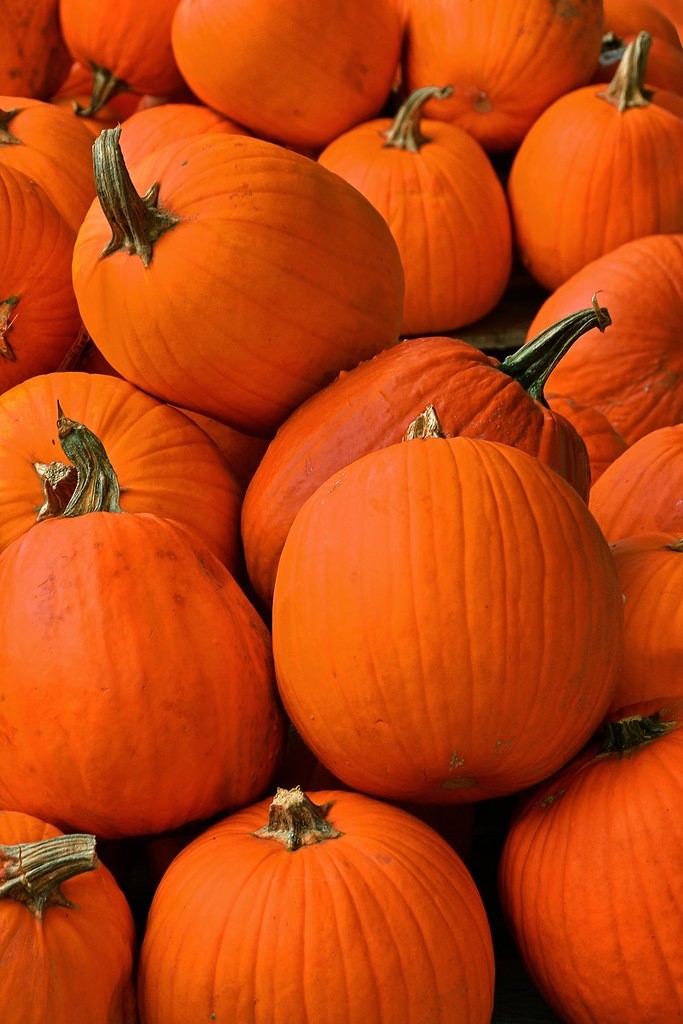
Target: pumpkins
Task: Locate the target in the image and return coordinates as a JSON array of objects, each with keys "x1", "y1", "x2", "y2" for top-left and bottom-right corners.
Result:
[{"x1": 0, "y1": 0, "x2": 683, "y2": 1024}]
